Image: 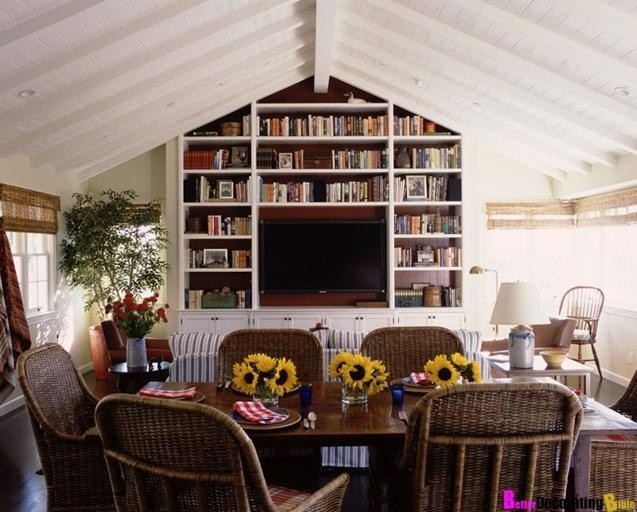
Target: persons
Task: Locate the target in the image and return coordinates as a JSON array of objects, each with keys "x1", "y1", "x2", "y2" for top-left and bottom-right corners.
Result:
[
  {"x1": 223, "y1": 186, "x2": 231, "y2": 197},
  {"x1": 409, "y1": 179, "x2": 421, "y2": 194}
]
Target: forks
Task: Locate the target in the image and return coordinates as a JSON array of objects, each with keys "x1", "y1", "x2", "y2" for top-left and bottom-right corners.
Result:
[{"x1": 398, "y1": 408, "x2": 408, "y2": 423}]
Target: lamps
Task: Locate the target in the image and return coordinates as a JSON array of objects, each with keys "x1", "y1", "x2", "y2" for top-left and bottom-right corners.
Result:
[
  {"x1": 470, "y1": 267, "x2": 499, "y2": 339},
  {"x1": 490, "y1": 281, "x2": 552, "y2": 370}
]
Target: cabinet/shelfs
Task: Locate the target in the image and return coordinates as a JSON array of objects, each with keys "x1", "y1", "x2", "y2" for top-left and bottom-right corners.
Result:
[
  {"x1": 253, "y1": 311, "x2": 324, "y2": 330},
  {"x1": 394, "y1": 310, "x2": 465, "y2": 329},
  {"x1": 179, "y1": 310, "x2": 248, "y2": 331},
  {"x1": 326, "y1": 311, "x2": 394, "y2": 330},
  {"x1": 164, "y1": 99, "x2": 465, "y2": 306}
]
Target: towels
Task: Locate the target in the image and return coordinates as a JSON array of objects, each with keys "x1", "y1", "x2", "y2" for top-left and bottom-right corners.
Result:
[
  {"x1": 410, "y1": 370, "x2": 433, "y2": 386},
  {"x1": 138, "y1": 385, "x2": 197, "y2": 399},
  {"x1": 232, "y1": 400, "x2": 289, "y2": 426}
]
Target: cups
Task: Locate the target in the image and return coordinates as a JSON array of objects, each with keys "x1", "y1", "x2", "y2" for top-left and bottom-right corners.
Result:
[
  {"x1": 299, "y1": 384, "x2": 313, "y2": 405},
  {"x1": 391, "y1": 384, "x2": 404, "y2": 404}
]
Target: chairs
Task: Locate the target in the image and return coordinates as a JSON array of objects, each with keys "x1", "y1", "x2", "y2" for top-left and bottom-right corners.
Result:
[
  {"x1": 17, "y1": 342, "x2": 111, "y2": 503},
  {"x1": 592, "y1": 371, "x2": 636, "y2": 511},
  {"x1": 93, "y1": 394, "x2": 349, "y2": 511},
  {"x1": 397, "y1": 381, "x2": 583, "y2": 511},
  {"x1": 361, "y1": 327, "x2": 466, "y2": 377},
  {"x1": 558, "y1": 286, "x2": 604, "y2": 386},
  {"x1": 216, "y1": 329, "x2": 324, "y2": 383}
]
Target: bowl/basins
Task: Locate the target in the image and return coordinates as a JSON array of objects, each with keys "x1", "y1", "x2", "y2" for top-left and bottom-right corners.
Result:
[{"x1": 539, "y1": 351, "x2": 569, "y2": 366}]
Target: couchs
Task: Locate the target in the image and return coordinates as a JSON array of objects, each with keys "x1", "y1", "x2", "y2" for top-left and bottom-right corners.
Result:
[
  {"x1": 170, "y1": 330, "x2": 485, "y2": 381},
  {"x1": 89, "y1": 319, "x2": 126, "y2": 381}
]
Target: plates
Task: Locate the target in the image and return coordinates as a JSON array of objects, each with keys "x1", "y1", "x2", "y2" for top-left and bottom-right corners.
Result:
[
  {"x1": 136, "y1": 391, "x2": 206, "y2": 403},
  {"x1": 401, "y1": 376, "x2": 436, "y2": 389},
  {"x1": 389, "y1": 378, "x2": 434, "y2": 393},
  {"x1": 224, "y1": 408, "x2": 301, "y2": 431},
  {"x1": 230, "y1": 408, "x2": 290, "y2": 425},
  {"x1": 231, "y1": 381, "x2": 301, "y2": 394}
]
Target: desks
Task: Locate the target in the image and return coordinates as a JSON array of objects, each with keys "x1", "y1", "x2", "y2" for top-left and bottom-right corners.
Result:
[
  {"x1": 110, "y1": 362, "x2": 168, "y2": 394},
  {"x1": 489, "y1": 349, "x2": 591, "y2": 376},
  {"x1": 494, "y1": 375, "x2": 636, "y2": 435},
  {"x1": 161, "y1": 381, "x2": 421, "y2": 438}
]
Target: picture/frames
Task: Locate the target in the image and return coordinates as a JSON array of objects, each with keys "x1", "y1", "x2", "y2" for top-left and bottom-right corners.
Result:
[
  {"x1": 202, "y1": 248, "x2": 228, "y2": 268},
  {"x1": 218, "y1": 181, "x2": 234, "y2": 199},
  {"x1": 406, "y1": 174, "x2": 427, "y2": 199},
  {"x1": 417, "y1": 250, "x2": 435, "y2": 263},
  {"x1": 278, "y1": 152, "x2": 293, "y2": 170}
]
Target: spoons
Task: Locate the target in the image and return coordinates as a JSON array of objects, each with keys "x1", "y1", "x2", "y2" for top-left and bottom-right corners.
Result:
[{"x1": 308, "y1": 411, "x2": 317, "y2": 430}]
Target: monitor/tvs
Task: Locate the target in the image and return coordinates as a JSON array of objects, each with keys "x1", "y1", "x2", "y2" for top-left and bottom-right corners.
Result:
[{"x1": 257, "y1": 218, "x2": 387, "y2": 294}]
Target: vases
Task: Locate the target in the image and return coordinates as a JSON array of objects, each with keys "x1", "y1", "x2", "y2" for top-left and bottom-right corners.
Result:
[
  {"x1": 342, "y1": 382, "x2": 368, "y2": 404},
  {"x1": 253, "y1": 391, "x2": 279, "y2": 412},
  {"x1": 126, "y1": 338, "x2": 147, "y2": 372}
]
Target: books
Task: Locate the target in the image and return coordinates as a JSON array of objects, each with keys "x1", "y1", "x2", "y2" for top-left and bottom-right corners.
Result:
[
  {"x1": 231, "y1": 249, "x2": 251, "y2": 268},
  {"x1": 257, "y1": 147, "x2": 276, "y2": 170},
  {"x1": 237, "y1": 290, "x2": 251, "y2": 308},
  {"x1": 331, "y1": 148, "x2": 389, "y2": 169},
  {"x1": 185, "y1": 248, "x2": 202, "y2": 268},
  {"x1": 394, "y1": 246, "x2": 412, "y2": 267},
  {"x1": 196, "y1": 175, "x2": 210, "y2": 202},
  {"x1": 236, "y1": 177, "x2": 252, "y2": 202},
  {"x1": 257, "y1": 176, "x2": 312, "y2": 203},
  {"x1": 394, "y1": 213, "x2": 460, "y2": 234},
  {"x1": 256, "y1": 114, "x2": 423, "y2": 136},
  {"x1": 207, "y1": 215, "x2": 252, "y2": 236},
  {"x1": 444, "y1": 288, "x2": 461, "y2": 307},
  {"x1": 326, "y1": 175, "x2": 388, "y2": 202},
  {"x1": 294, "y1": 150, "x2": 304, "y2": 168},
  {"x1": 395, "y1": 178, "x2": 405, "y2": 202},
  {"x1": 435, "y1": 247, "x2": 462, "y2": 268},
  {"x1": 184, "y1": 146, "x2": 249, "y2": 169},
  {"x1": 425, "y1": 132, "x2": 451, "y2": 136},
  {"x1": 428, "y1": 176, "x2": 448, "y2": 201}
]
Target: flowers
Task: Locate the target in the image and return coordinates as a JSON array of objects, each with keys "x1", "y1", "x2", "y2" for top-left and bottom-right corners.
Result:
[
  {"x1": 231, "y1": 353, "x2": 298, "y2": 395},
  {"x1": 328, "y1": 351, "x2": 389, "y2": 396},
  {"x1": 424, "y1": 353, "x2": 480, "y2": 387},
  {"x1": 106, "y1": 293, "x2": 167, "y2": 338}
]
